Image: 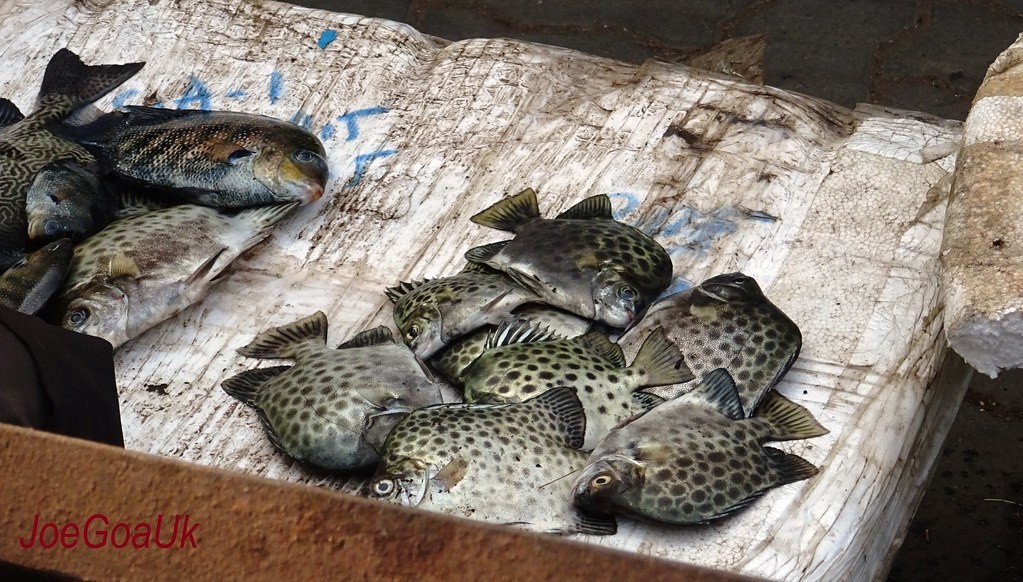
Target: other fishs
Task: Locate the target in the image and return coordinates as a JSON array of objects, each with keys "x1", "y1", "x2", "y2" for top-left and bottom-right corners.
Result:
[{"x1": 1, "y1": 47, "x2": 829, "y2": 541}]
